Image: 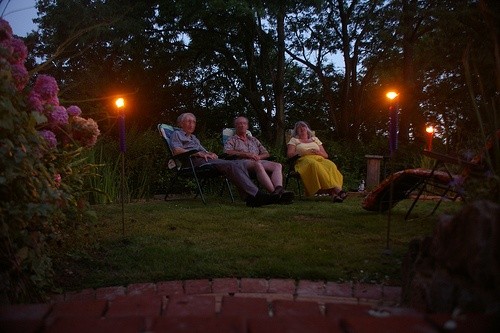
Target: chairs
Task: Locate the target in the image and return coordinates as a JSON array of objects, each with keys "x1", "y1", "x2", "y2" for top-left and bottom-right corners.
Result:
[
  {"x1": 283, "y1": 129, "x2": 339, "y2": 202},
  {"x1": 219, "y1": 128, "x2": 279, "y2": 200},
  {"x1": 158, "y1": 124, "x2": 235, "y2": 206},
  {"x1": 358, "y1": 128, "x2": 500, "y2": 224}
]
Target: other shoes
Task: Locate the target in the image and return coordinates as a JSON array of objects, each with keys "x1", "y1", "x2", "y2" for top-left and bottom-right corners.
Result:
[
  {"x1": 271, "y1": 192, "x2": 295, "y2": 204},
  {"x1": 333, "y1": 196, "x2": 342, "y2": 203},
  {"x1": 246, "y1": 192, "x2": 278, "y2": 206},
  {"x1": 276, "y1": 188, "x2": 295, "y2": 202},
  {"x1": 338, "y1": 191, "x2": 347, "y2": 199}
]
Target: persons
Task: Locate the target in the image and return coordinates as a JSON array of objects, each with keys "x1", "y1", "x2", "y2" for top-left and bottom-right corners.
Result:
[
  {"x1": 286, "y1": 121, "x2": 349, "y2": 203},
  {"x1": 221, "y1": 116, "x2": 295, "y2": 205},
  {"x1": 169, "y1": 113, "x2": 274, "y2": 208}
]
